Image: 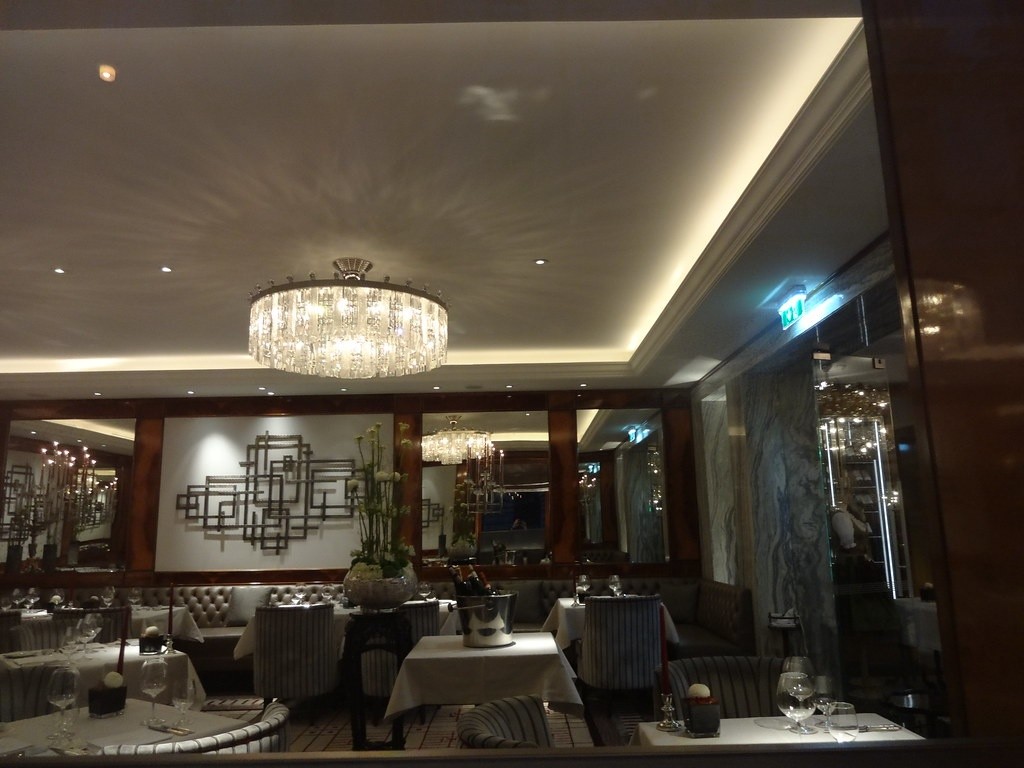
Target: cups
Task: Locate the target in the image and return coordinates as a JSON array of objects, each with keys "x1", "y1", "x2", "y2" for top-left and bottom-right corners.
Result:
[
  {"x1": 139, "y1": 633, "x2": 169, "y2": 656},
  {"x1": 576, "y1": 591, "x2": 590, "y2": 606},
  {"x1": 827, "y1": 702, "x2": 859, "y2": 743},
  {"x1": 679, "y1": 696, "x2": 722, "y2": 738},
  {"x1": 426, "y1": 587, "x2": 437, "y2": 602}
]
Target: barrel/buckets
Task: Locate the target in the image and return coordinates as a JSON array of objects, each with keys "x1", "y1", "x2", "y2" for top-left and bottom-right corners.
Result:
[{"x1": 448, "y1": 589, "x2": 519, "y2": 648}]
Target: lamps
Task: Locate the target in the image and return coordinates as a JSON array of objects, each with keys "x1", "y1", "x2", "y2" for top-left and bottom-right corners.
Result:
[
  {"x1": 422, "y1": 415, "x2": 494, "y2": 464},
  {"x1": 249, "y1": 259, "x2": 448, "y2": 379},
  {"x1": 812, "y1": 360, "x2": 887, "y2": 452}
]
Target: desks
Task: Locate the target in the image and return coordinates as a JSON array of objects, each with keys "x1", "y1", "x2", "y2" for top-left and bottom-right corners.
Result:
[{"x1": 0, "y1": 640, "x2": 244, "y2": 755}]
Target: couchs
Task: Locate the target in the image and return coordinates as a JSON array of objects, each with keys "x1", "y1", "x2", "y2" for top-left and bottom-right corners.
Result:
[{"x1": 0, "y1": 580, "x2": 754, "y2": 655}]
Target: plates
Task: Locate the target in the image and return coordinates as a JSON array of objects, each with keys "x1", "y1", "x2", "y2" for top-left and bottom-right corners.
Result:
[
  {"x1": 4, "y1": 651, "x2": 37, "y2": 658},
  {"x1": 87, "y1": 728, "x2": 174, "y2": 748},
  {"x1": 0, "y1": 737, "x2": 34, "y2": 756}
]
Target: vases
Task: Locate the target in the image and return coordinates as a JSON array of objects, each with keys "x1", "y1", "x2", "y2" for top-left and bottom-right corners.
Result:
[{"x1": 345, "y1": 570, "x2": 415, "y2": 606}]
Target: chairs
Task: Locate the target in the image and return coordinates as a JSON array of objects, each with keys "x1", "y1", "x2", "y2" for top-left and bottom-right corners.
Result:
[
  {"x1": 100, "y1": 702, "x2": 290, "y2": 752},
  {"x1": 576, "y1": 595, "x2": 786, "y2": 719},
  {"x1": 11, "y1": 618, "x2": 76, "y2": 649},
  {"x1": 253, "y1": 605, "x2": 333, "y2": 699},
  {"x1": 88, "y1": 607, "x2": 129, "y2": 642},
  {"x1": 456, "y1": 695, "x2": 553, "y2": 746}
]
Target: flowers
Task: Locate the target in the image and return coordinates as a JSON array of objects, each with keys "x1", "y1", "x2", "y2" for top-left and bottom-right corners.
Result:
[{"x1": 348, "y1": 426, "x2": 415, "y2": 566}]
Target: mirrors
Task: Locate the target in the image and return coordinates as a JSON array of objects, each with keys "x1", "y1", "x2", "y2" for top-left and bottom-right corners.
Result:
[
  {"x1": 420, "y1": 411, "x2": 669, "y2": 566},
  {"x1": 0, "y1": 418, "x2": 135, "y2": 567},
  {"x1": 809, "y1": 332, "x2": 944, "y2": 703}
]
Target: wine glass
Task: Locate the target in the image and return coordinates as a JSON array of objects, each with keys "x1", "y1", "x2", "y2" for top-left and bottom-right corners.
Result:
[
  {"x1": 579, "y1": 574, "x2": 624, "y2": 596},
  {"x1": 1, "y1": 585, "x2": 142, "y2": 615},
  {"x1": 418, "y1": 580, "x2": 432, "y2": 603},
  {"x1": 291, "y1": 582, "x2": 336, "y2": 605},
  {"x1": 60, "y1": 613, "x2": 104, "y2": 665},
  {"x1": 46, "y1": 667, "x2": 81, "y2": 739},
  {"x1": 775, "y1": 656, "x2": 838, "y2": 735},
  {"x1": 139, "y1": 657, "x2": 196, "y2": 729}
]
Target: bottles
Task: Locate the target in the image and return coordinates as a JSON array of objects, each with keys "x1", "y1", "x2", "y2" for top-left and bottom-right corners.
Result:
[{"x1": 447, "y1": 562, "x2": 499, "y2": 595}]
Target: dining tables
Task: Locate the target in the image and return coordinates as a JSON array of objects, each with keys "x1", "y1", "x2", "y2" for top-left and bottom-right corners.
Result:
[
  {"x1": 639, "y1": 713, "x2": 929, "y2": 745},
  {"x1": 0, "y1": 699, "x2": 245, "y2": 757},
  {"x1": 132, "y1": 606, "x2": 204, "y2": 644},
  {"x1": 385, "y1": 633, "x2": 583, "y2": 718}
]
